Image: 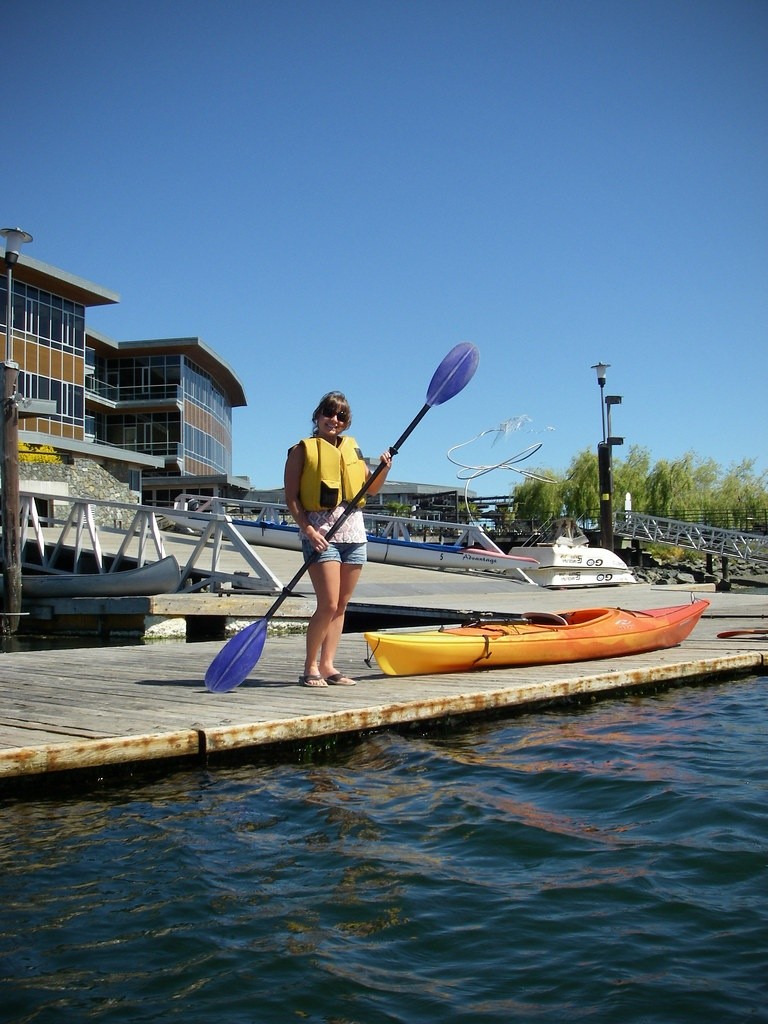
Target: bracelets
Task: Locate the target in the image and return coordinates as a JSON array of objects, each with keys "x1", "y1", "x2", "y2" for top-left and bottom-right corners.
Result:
[{"x1": 301, "y1": 522, "x2": 310, "y2": 532}]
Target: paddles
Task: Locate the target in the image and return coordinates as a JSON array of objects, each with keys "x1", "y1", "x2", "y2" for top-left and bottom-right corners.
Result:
[{"x1": 202, "y1": 341, "x2": 483, "y2": 697}]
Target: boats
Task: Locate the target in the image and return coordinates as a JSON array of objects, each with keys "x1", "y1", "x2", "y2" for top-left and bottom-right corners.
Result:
[
  {"x1": 160, "y1": 513, "x2": 541, "y2": 569},
  {"x1": 505, "y1": 546, "x2": 637, "y2": 590},
  {"x1": 1, "y1": 555, "x2": 181, "y2": 598},
  {"x1": 362, "y1": 598, "x2": 714, "y2": 677}
]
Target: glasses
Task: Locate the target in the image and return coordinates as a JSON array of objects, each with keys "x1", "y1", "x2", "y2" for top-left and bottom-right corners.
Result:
[{"x1": 323, "y1": 407, "x2": 349, "y2": 422}]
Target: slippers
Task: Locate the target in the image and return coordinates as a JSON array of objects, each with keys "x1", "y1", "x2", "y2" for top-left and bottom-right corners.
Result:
[
  {"x1": 325, "y1": 672, "x2": 357, "y2": 688},
  {"x1": 299, "y1": 671, "x2": 329, "y2": 689}
]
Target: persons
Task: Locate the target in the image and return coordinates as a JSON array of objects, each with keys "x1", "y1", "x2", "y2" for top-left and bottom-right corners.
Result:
[{"x1": 284, "y1": 392, "x2": 392, "y2": 687}]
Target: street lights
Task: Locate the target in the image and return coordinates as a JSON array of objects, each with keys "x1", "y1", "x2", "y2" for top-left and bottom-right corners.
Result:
[{"x1": 590, "y1": 361, "x2": 623, "y2": 552}]
